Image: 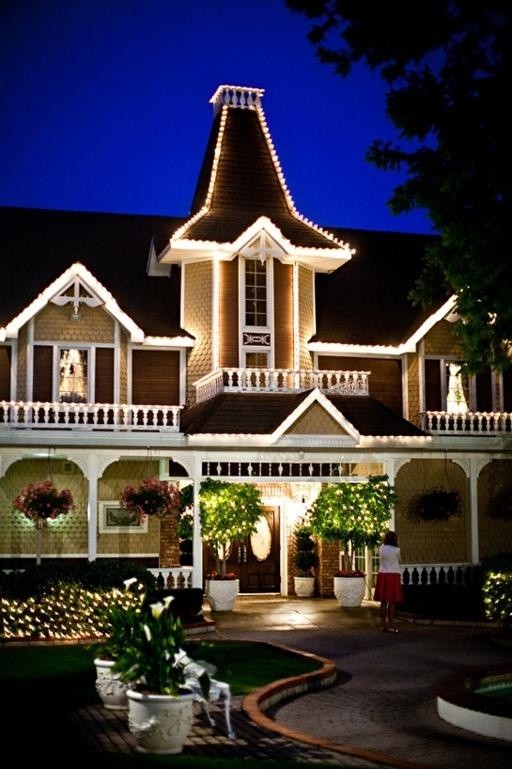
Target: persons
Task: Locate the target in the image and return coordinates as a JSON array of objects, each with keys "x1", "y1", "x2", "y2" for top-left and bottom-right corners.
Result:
[{"x1": 373, "y1": 531, "x2": 404, "y2": 633}]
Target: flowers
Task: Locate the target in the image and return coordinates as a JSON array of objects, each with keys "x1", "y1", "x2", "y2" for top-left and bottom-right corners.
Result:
[
  {"x1": 120, "y1": 475, "x2": 182, "y2": 524},
  {"x1": 12, "y1": 480, "x2": 76, "y2": 529}
]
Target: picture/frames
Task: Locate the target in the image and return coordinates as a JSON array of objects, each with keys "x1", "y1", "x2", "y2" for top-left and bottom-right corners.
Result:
[{"x1": 97, "y1": 500, "x2": 149, "y2": 534}]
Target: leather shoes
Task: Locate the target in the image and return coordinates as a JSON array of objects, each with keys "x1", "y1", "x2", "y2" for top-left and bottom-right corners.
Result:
[{"x1": 380, "y1": 627, "x2": 400, "y2": 631}]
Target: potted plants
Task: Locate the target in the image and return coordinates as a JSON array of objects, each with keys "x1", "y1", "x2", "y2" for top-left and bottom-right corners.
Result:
[
  {"x1": 93, "y1": 604, "x2": 196, "y2": 755},
  {"x1": 293, "y1": 472, "x2": 399, "y2": 608},
  {"x1": 173, "y1": 477, "x2": 265, "y2": 611}
]
